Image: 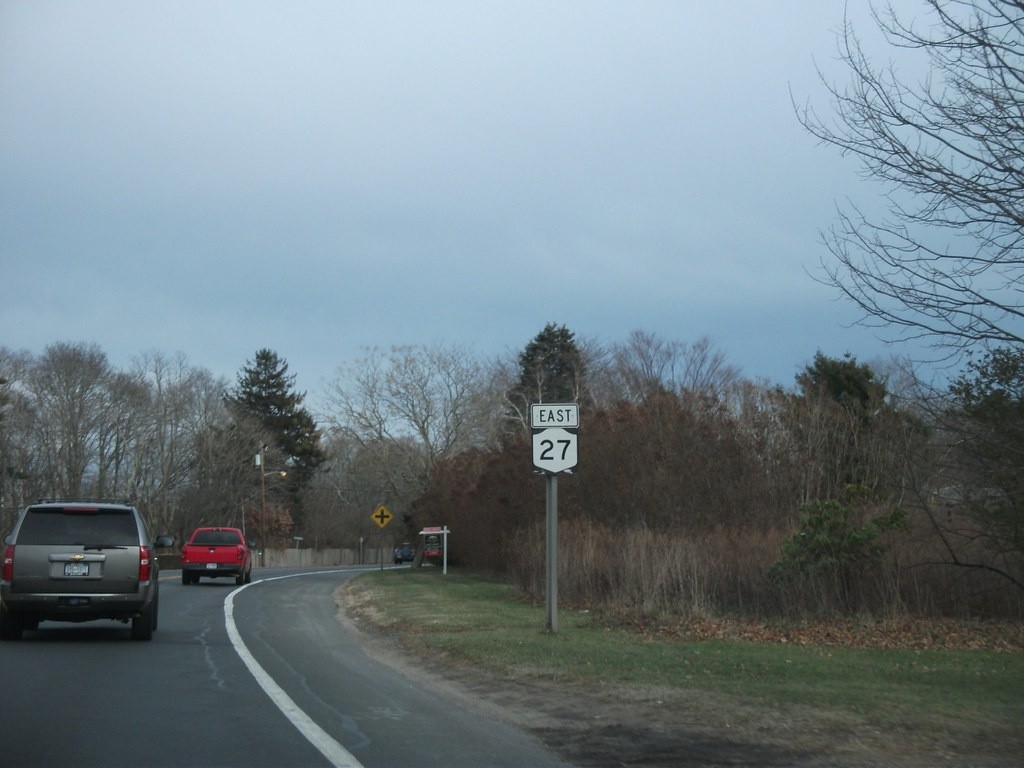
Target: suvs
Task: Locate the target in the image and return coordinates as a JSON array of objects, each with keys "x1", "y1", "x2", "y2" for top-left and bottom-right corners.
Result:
[
  {"x1": 0, "y1": 501, "x2": 160, "y2": 641},
  {"x1": 393, "y1": 542, "x2": 416, "y2": 565}
]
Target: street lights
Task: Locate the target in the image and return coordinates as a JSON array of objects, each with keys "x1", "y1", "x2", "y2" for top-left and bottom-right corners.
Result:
[{"x1": 259, "y1": 440, "x2": 287, "y2": 567}]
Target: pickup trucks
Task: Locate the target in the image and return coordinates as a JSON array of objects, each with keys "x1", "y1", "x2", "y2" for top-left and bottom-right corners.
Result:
[{"x1": 179, "y1": 526, "x2": 253, "y2": 585}]
